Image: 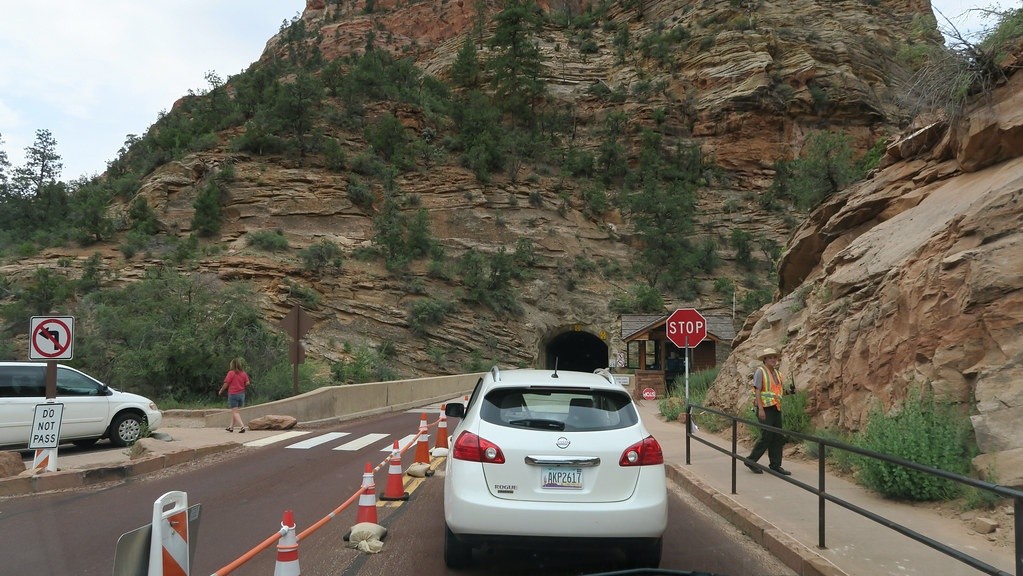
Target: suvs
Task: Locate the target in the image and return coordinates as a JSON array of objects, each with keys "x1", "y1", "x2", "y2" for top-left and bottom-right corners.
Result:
[{"x1": 443, "y1": 366, "x2": 671, "y2": 569}]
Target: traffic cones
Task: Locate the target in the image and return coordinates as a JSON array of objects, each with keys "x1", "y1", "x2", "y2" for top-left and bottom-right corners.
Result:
[
  {"x1": 464, "y1": 395, "x2": 469, "y2": 414},
  {"x1": 341, "y1": 463, "x2": 377, "y2": 542},
  {"x1": 379, "y1": 441, "x2": 410, "y2": 502},
  {"x1": 406, "y1": 412, "x2": 435, "y2": 477},
  {"x1": 429, "y1": 404, "x2": 449, "y2": 455},
  {"x1": 273, "y1": 510, "x2": 301, "y2": 576}
]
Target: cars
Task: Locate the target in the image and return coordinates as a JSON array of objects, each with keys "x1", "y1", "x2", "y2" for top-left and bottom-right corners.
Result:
[{"x1": 0, "y1": 362, "x2": 163, "y2": 451}]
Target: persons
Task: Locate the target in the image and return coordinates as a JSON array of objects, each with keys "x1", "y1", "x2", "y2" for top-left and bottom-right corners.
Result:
[
  {"x1": 219, "y1": 359, "x2": 250, "y2": 433},
  {"x1": 664, "y1": 352, "x2": 681, "y2": 394},
  {"x1": 744, "y1": 348, "x2": 792, "y2": 475}
]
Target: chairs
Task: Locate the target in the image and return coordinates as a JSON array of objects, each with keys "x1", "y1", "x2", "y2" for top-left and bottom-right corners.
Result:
[
  {"x1": 500, "y1": 394, "x2": 533, "y2": 424},
  {"x1": 567, "y1": 399, "x2": 603, "y2": 428}
]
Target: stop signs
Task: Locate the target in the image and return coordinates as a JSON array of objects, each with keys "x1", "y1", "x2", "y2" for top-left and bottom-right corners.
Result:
[
  {"x1": 667, "y1": 309, "x2": 707, "y2": 348},
  {"x1": 643, "y1": 388, "x2": 655, "y2": 400}
]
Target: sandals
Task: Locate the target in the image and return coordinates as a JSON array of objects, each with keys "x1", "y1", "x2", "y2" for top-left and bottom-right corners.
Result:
[
  {"x1": 226, "y1": 427, "x2": 233, "y2": 432},
  {"x1": 239, "y1": 427, "x2": 245, "y2": 433}
]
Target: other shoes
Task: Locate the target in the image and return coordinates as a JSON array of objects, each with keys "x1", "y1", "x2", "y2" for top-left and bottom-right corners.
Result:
[
  {"x1": 777, "y1": 467, "x2": 791, "y2": 475},
  {"x1": 744, "y1": 462, "x2": 763, "y2": 473}
]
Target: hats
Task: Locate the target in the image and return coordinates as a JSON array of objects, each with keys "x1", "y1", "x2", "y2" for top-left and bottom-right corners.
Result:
[{"x1": 757, "y1": 348, "x2": 780, "y2": 361}]
